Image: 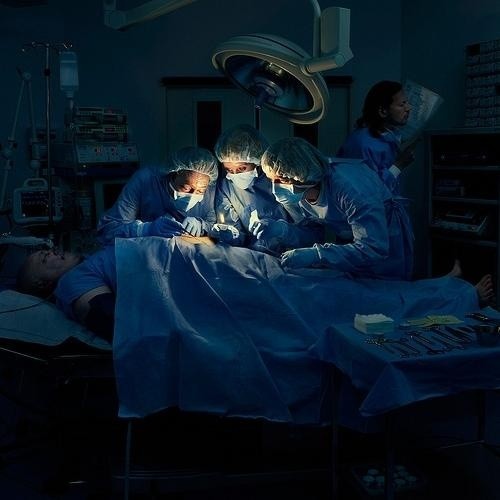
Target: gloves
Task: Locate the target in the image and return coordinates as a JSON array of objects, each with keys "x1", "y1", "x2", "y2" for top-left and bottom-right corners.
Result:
[
  {"x1": 248, "y1": 218, "x2": 288, "y2": 241},
  {"x1": 279, "y1": 246, "x2": 320, "y2": 268},
  {"x1": 210, "y1": 223, "x2": 240, "y2": 243},
  {"x1": 179, "y1": 216, "x2": 202, "y2": 239},
  {"x1": 141, "y1": 212, "x2": 183, "y2": 239}
]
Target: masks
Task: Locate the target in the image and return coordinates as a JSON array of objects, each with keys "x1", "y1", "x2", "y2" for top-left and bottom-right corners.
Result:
[
  {"x1": 225, "y1": 166, "x2": 258, "y2": 190},
  {"x1": 169, "y1": 182, "x2": 207, "y2": 214},
  {"x1": 270, "y1": 182, "x2": 311, "y2": 208}
]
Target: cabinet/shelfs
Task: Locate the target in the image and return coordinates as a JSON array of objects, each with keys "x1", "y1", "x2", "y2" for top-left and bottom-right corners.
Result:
[
  {"x1": 425, "y1": 126, "x2": 499, "y2": 311},
  {"x1": 320, "y1": 308, "x2": 498, "y2": 499}
]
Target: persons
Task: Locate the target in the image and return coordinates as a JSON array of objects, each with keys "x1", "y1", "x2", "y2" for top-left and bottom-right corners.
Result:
[
  {"x1": 203, "y1": 129, "x2": 289, "y2": 257},
  {"x1": 93, "y1": 146, "x2": 221, "y2": 246},
  {"x1": 335, "y1": 80, "x2": 415, "y2": 196},
  {"x1": 247, "y1": 135, "x2": 416, "y2": 284},
  {"x1": 17, "y1": 243, "x2": 494, "y2": 346}
]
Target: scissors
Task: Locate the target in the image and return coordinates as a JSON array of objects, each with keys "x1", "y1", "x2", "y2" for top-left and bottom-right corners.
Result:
[{"x1": 365, "y1": 328, "x2": 470, "y2": 360}]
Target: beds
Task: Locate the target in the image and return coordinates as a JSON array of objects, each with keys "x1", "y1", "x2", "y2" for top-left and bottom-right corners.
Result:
[{"x1": 0, "y1": 289, "x2": 500, "y2": 499}]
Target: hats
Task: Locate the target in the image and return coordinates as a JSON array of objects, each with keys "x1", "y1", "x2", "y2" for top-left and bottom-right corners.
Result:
[
  {"x1": 213, "y1": 123, "x2": 269, "y2": 168},
  {"x1": 259, "y1": 135, "x2": 330, "y2": 185},
  {"x1": 157, "y1": 145, "x2": 220, "y2": 184}
]
